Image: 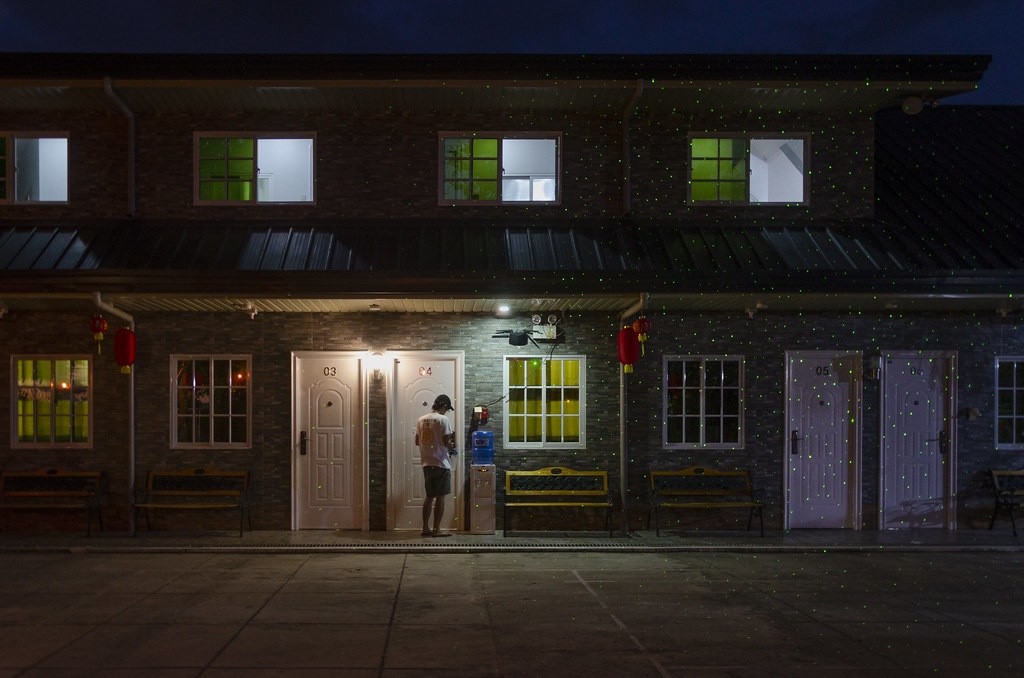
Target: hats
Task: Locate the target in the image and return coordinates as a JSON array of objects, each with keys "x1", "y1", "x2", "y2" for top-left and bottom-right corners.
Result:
[{"x1": 435, "y1": 394, "x2": 454, "y2": 410}]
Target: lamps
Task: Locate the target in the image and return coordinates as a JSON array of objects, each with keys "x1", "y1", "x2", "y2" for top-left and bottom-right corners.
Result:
[{"x1": 531, "y1": 313, "x2": 557, "y2": 340}]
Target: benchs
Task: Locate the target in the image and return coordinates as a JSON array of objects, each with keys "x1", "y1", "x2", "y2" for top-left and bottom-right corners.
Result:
[
  {"x1": 647, "y1": 464, "x2": 767, "y2": 538},
  {"x1": 0, "y1": 466, "x2": 105, "y2": 537},
  {"x1": 131, "y1": 467, "x2": 252, "y2": 538},
  {"x1": 502, "y1": 467, "x2": 615, "y2": 537},
  {"x1": 988, "y1": 467, "x2": 1024, "y2": 536}
]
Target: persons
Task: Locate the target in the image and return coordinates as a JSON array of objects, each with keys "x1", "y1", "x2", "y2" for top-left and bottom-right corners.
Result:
[{"x1": 415, "y1": 394, "x2": 454, "y2": 536}]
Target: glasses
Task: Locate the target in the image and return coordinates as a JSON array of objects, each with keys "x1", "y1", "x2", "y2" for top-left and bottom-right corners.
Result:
[{"x1": 446, "y1": 408, "x2": 451, "y2": 412}]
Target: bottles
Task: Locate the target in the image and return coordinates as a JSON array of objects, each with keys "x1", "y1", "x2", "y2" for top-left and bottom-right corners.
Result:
[{"x1": 471, "y1": 431, "x2": 494, "y2": 464}]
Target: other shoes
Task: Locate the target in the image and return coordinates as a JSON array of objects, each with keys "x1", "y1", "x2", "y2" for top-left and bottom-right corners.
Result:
[
  {"x1": 431, "y1": 528, "x2": 453, "y2": 537},
  {"x1": 421, "y1": 528, "x2": 432, "y2": 536}
]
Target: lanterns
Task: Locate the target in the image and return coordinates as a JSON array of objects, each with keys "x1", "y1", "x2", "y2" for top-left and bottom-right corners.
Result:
[
  {"x1": 634, "y1": 316, "x2": 653, "y2": 355},
  {"x1": 115, "y1": 326, "x2": 136, "y2": 374},
  {"x1": 90, "y1": 314, "x2": 108, "y2": 354},
  {"x1": 618, "y1": 326, "x2": 639, "y2": 373}
]
